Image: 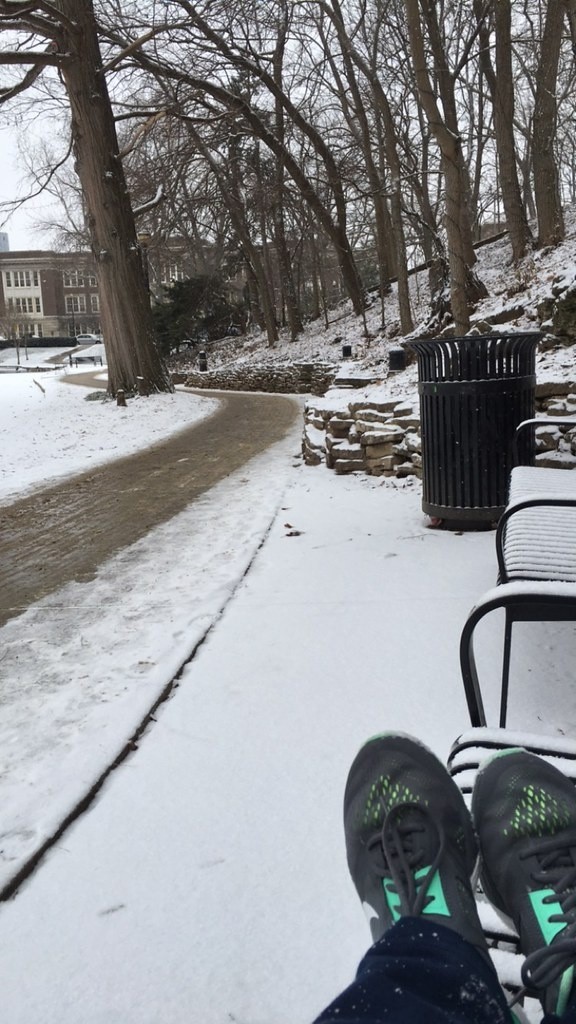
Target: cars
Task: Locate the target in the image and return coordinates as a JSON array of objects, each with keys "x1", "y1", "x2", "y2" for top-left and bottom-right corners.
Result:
[
  {"x1": 137, "y1": 229, "x2": 152, "y2": 309},
  {"x1": 76, "y1": 334, "x2": 102, "y2": 345}
]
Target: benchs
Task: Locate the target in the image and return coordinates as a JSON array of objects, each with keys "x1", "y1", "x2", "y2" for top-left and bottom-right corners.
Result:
[
  {"x1": 69, "y1": 356, "x2": 102, "y2": 367},
  {"x1": 495, "y1": 417, "x2": 576, "y2": 729},
  {"x1": 441, "y1": 579, "x2": 576, "y2": 1024}
]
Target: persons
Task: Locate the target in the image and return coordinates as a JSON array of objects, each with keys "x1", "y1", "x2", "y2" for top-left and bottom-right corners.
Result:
[{"x1": 318, "y1": 733, "x2": 576, "y2": 1024}]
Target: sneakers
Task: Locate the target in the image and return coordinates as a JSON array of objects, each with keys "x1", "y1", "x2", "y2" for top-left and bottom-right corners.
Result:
[
  {"x1": 472, "y1": 747, "x2": 576, "y2": 1022},
  {"x1": 340, "y1": 732, "x2": 533, "y2": 1022}
]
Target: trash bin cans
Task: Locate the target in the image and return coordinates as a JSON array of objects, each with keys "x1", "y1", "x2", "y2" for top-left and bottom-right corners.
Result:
[{"x1": 405, "y1": 331, "x2": 547, "y2": 531}]
